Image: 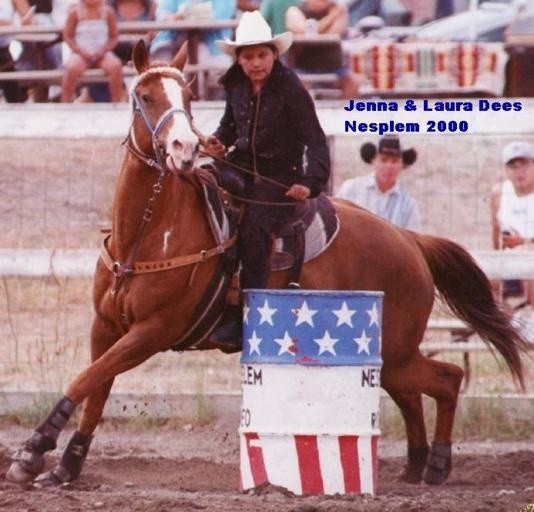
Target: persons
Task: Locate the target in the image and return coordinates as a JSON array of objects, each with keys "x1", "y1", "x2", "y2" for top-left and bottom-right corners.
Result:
[
  {"x1": 480, "y1": 140, "x2": 534, "y2": 346},
  {"x1": 336, "y1": 132, "x2": 422, "y2": 234},
  {"x1": 201, "y1": 9, "x2": 330, "y2": 346}
]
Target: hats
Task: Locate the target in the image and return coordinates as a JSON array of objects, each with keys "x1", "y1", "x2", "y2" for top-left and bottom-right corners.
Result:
[
  {"x1": 360, "y1": 135, "x2": 417, "y2": 164},
  {"x1": 504, "y1": 143, "x2": 534, "y2": 164},
  {"x1": 214, "y1": 11, "x2": 293, "y2": 56}
]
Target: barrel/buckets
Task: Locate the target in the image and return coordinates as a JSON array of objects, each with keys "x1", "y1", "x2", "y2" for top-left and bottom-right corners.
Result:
[{"x1": 239, "y1": 285, "x2": 387, "y2": 502}]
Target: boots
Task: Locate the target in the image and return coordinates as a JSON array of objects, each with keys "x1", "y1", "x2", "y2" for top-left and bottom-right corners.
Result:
[{"x1": 209, "y1": 223, "x2": 274, "y2": 344}]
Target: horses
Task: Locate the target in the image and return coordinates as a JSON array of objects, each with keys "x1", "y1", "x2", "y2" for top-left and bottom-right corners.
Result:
[{"x1": 4, "y1": 37, "x2": 534, "y2": 490}]
